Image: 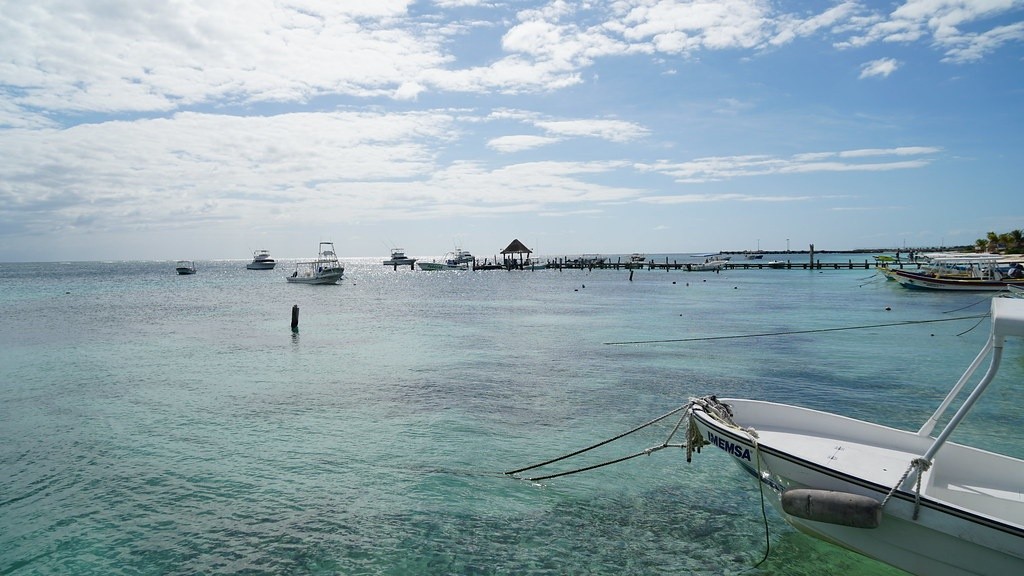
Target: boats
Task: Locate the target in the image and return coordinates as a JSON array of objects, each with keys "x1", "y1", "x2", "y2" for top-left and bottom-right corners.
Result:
[
  {"x1": 748, "y1": 255, "x2": 763, "y2": 260},
  {"x1": 683, "y1": 298, "x2": 1024, "y2": 576},
  {"x1": 768, "y1": 260, "x2": 786, "y2": 267},
  {"x1": 375, "y1": 235, "x2": 418, "y2": 266},
  {"x1": 285, "y1": 241, "x2": 346, "y2": 287},
  {"x1": 575, "y1": 251, "x2": 607, "y2": 266},
  {"x1": 632, "y1": 256, "x2": 645, "y2": 261},
  {"x1": 687, "y1": 252, "x2": 728, "y2": 273},
  {"x1": 873, "y1": 253, "x2": 1024, "y2": 293},
  {"x1": 415, "y1": 255, "x2": 467, "y2": 275},
  {"x1": 245, "y1": 249, "x2": 277, "y2": 271},
  {"x1": 720, "y1": 257, "x2": 731, "y2": 260},
  {"x1": 442, "y1": 241, "x2": 475, "y2": 267},
  {"x1": 174, "y1": 259, "x2": 199, "y2": 278}
]
